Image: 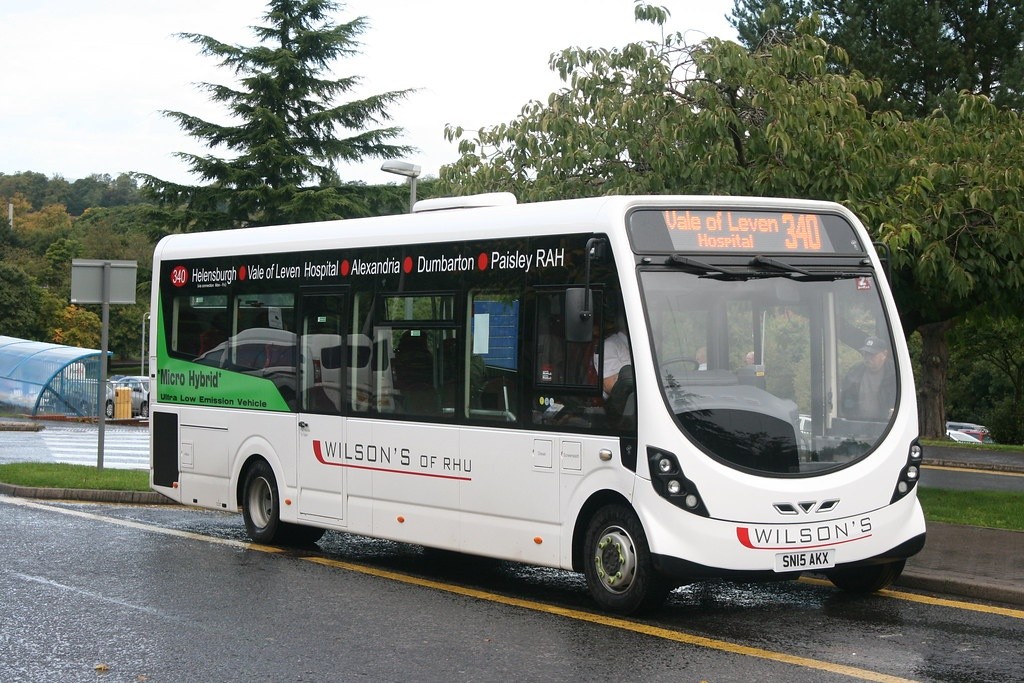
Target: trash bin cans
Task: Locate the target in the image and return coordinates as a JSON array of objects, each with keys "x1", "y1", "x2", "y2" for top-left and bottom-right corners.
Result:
[{"x1": 115, "y1": 386, "x2": 132, "y2": 420}]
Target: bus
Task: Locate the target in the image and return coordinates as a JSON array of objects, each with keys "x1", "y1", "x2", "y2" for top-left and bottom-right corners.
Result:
[
  {"x1": 189, "y1": 329, "x2": 397, "y2": 417},
  {"x1": 146, "y1": 187, "x2": 929, "y2": 614}
]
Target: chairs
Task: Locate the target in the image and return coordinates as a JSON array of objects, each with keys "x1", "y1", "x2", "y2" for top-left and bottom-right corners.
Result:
[
  {"x1": 582, "y1": 322, "x2": 620, "y2": 406},
  {"x1": 196, "y1": 330, "x2": 295, "y2": 368}
]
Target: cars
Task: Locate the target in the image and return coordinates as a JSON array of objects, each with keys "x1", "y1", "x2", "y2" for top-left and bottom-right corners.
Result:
[
  {"x1": 116, "y1": 376, "x2": 149, "y2": 415},
  {"x1": 799, "y1": 414, "x2": 813, "y2": 433},
  {"x1": 51, "y1": 378, "x2": 118, "y2": 418},
  {"x1": 946, "y1": 422, "x2": 994, "y2": 444}
]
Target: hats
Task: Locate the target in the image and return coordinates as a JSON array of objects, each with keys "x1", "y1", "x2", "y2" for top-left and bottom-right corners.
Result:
[{"x1": 859, "y1": 336, "x2": 887, "y2": 355}]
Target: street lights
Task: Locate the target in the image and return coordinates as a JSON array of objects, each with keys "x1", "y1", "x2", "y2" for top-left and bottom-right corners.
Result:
[
  {"x1": 141, "y1": 312, "x2": 150, "y2": 375},
  {"x1": 382, "y1": 162, "x2": 420, "y2": 322}
]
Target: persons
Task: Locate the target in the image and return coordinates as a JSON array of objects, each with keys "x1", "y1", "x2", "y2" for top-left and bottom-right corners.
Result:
[
  {"x1": 590, "y1": 312, "x2": 636, "y2": 401},
  {"x1": 744, "y1": 351, "x2": 754, "y2": 365},
  {"x1": 695, "y1": 346, "x2": 707, "y2": 370},
  {"x1": 838, "y1": 335, "x2": 897, "y2": 424}
]
